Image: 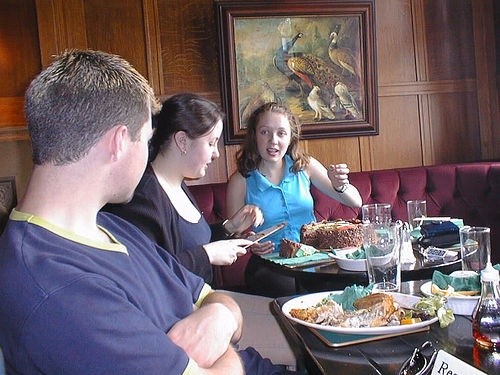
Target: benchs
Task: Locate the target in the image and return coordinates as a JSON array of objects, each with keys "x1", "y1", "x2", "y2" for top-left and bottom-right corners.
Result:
[{"x1": 177, "y1": 160, "x2": 500, "y2": 304}]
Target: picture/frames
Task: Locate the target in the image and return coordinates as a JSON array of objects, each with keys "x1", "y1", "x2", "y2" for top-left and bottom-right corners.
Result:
[{"x1": 216, "y1": 1, "x2": 379, "y2": 146}]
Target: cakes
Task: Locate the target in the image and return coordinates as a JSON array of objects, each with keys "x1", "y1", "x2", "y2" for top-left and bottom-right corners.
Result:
[
  {"x1": 300, "y1": 218, "x2": 376, "y2": 249},
  {"x1": 278, "y1": 238, "x2": 318, "y2": 258}
]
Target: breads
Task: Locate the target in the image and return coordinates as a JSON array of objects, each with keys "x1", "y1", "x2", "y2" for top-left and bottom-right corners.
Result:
[{"x1": 289, "y1": 292, "x2": 398, "y2": 328}]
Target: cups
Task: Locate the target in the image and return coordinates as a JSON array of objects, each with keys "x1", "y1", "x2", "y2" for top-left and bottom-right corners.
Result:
[
  {"x1": 459, "y1": 226, "x2": 490, "y2": 275},
  {"x1": 361, "y1": 203, "x2": 392, "y2": 225},
  {"x1": 407, "y1": 199, "x2": 427, "y2": 230},
  {"x1": 361, "y1": 221, "x2": 403, "y2": 294}
]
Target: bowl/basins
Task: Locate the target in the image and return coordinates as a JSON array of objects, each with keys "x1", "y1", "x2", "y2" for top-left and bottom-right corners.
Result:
[
  {"x1": 329, "y1": 247, "x2": 368, "y2": 272},
  {"x1": 419, "y1": 280, "x2": 480, "y2": 316}
]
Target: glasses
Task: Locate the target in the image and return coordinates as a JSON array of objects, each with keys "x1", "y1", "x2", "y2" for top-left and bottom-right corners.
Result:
[{"x1": 397, "y1": 340, "x2": 439, "y2": 375}]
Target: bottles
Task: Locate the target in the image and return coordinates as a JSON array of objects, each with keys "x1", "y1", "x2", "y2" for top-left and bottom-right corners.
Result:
[
  {"x1": 396, "y1": 220, "x2": 416, "y2": 264},
  {"x1": 471, "y1": 263, "x2": 500, "y2": 350}
]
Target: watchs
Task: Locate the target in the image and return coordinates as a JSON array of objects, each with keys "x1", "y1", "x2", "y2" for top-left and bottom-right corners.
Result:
[
  {"x1": 221, "y1": 218, "x2": 236, "y2": 236},
  {"x1": 333, "y1": 184, "x2": 347, "y2": 193}
]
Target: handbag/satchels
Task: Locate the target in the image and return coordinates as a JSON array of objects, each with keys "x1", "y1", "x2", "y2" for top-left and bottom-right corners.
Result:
[{"x1": 417, "y1": 219, "x2": 468, "y2": 251}]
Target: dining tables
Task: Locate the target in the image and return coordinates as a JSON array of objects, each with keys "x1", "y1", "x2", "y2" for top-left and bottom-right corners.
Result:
[
  {"x1": 257, "y1": 218, "x2": 500, "y2": 375},
  {"x1": 270, "y1": 270, "x2": 499, "y2": 374}
]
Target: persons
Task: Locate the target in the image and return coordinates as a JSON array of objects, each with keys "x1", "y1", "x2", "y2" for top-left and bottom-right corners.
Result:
[
  {"x1": 100, "y1": 93, "x2": 264, "y2": 289},
  {"x1": 225, "y1": 102, "x2": 363, "y2": 298},
  {"x1": 0, "y1": 48, "x2": 308, "y2": 375}
]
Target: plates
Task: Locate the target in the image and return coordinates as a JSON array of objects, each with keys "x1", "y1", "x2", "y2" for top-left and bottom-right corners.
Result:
[{"x1": 280, "y1": 290, "x2": 440, "y2": 336}]
